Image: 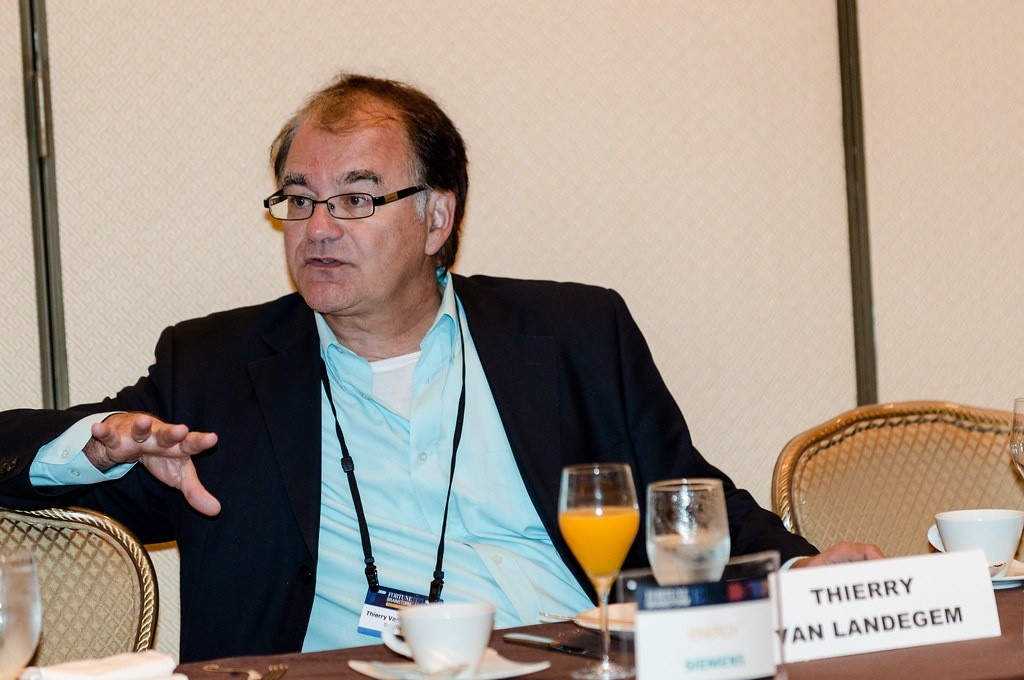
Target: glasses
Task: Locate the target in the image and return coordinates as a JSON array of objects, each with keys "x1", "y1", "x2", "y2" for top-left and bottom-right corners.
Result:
[{"x1": 262, "y1": 181, "x2": 437, "y2": 220}]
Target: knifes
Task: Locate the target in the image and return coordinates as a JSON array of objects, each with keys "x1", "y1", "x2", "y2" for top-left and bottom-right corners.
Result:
[{"x1": 502, "y1": 632, "x2": 612, "y2": 660}]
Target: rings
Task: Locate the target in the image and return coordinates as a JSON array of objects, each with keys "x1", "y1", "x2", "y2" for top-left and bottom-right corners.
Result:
[{"x1": 135, "y1": 440, "x2": 144, "y2": 443}]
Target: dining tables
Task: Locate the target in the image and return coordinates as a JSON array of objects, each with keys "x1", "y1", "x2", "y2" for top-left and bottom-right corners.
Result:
[{"x1": 170, "y1": 582, "x2": 1023, "y2": 679}]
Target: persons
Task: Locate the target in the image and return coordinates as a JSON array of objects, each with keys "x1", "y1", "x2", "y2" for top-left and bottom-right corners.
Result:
[{"x1": 0, "y1": 75, "x2": 887, "y2": 667}]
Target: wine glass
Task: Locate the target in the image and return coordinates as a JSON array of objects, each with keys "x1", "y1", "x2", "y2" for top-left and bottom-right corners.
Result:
[{"x1": 557, "y1": 465, "x2": 642, "y2": 680}]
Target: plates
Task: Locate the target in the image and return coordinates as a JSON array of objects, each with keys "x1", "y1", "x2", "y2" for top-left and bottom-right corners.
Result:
[
  {"x1": 574, "y1": 602, "x2": 636, "y2": 640},
  {"x1": 347, "y1": 646, "x2": 550, "y2": 680},
  {"x1": 991, "y1": 558, "x2": 1024, "y2": 590}
]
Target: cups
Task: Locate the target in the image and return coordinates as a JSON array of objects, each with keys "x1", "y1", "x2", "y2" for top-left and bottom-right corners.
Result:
[
  {"x1": 935, "y1": 508, "x2": 1024, "y2": 576},
  {"x1": 382, "y1": 603, "x2": 495, "y2": 678},
  {"x1": 646, "y1": 478, "x2": 731, "y2": 587},
  {"x1": 0, "y1": 554, "x2": 42, "y2": 680}
]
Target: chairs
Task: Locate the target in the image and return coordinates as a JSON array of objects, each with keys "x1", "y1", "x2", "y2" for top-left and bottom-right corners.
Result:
[
  {"x1": 0, "y1": 504, "x2": 161, "y2": 669},
  {"x1": 770, "y1": 398, "x2": 1024, "y2": 560}
]
